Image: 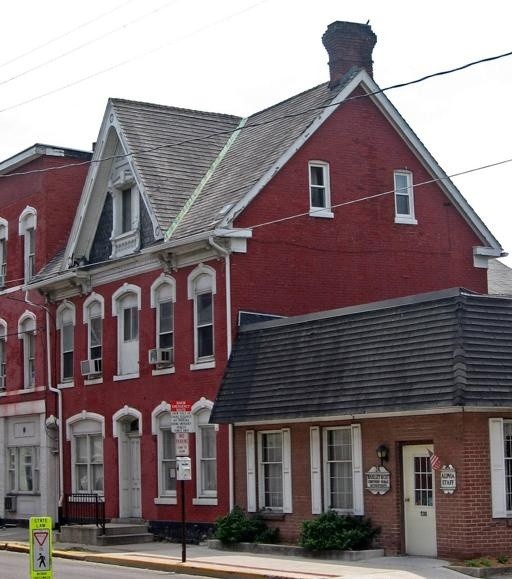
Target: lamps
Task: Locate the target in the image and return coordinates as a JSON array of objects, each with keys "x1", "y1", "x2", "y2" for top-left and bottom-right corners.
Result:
[{"x1": 374, "y1": 442, "x2": 389, "y2": 467}]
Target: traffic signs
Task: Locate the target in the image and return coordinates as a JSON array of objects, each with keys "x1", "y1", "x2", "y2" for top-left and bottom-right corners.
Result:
[
  {"x1": 174, "y1": 433, "x2": 189, "y2": 457},
  {"x1": 170, "y1": 399, "x2": 192, "y2": 412},
  {"x1": 171, "y1": 413, "x2": 193, "y2": 432}
]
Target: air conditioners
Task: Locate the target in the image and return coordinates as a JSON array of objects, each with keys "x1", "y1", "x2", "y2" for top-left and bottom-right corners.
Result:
[
  {"x1": 148, "y1": 348, "x2": 173, "y2": 365},
  {"x1": 79, "y1": 358, "x2": 102, "y2": 376}
]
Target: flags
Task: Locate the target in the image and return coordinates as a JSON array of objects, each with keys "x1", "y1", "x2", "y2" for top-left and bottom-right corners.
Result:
[{"x1": 428, "y1": 450, "x2": 441, "y2": 470}]
